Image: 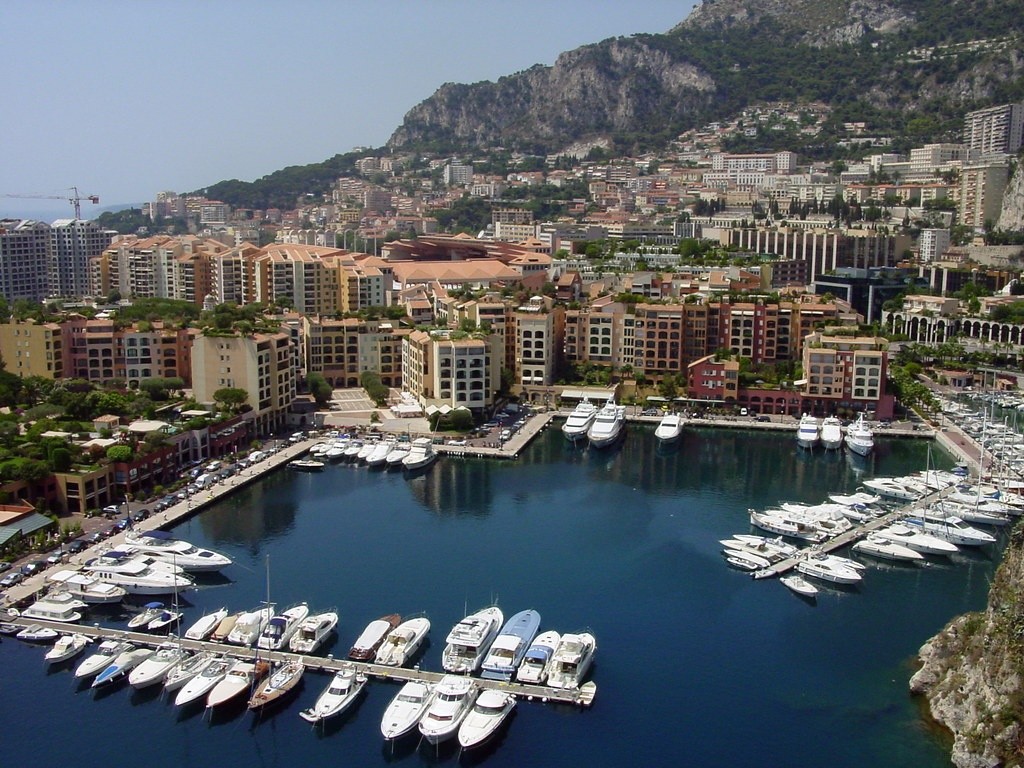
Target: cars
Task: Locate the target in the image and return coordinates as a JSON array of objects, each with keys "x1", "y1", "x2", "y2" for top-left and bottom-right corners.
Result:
[
  {"x1": 840, "y1": 417, "x2": 939, "y2": 431},
  {"x1": 1, "y1": 428, "x2": 384, "y2": 586},
  {"x1": 385, "y1": 397, "x2": 773, "y2": 448}
]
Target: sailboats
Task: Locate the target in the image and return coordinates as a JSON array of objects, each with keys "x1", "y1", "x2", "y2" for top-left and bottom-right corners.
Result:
[
  {"x1": 161, "y1": 549, "x2": 217, "y2": 694},
  {"x1": 246, "y1": 552, "x2": 307, "y2": 719},
  {"x1": 922, "y1": 439, "x2": 965, "y2": 544}
]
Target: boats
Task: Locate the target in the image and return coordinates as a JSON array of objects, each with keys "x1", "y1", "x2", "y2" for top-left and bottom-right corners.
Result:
[
  {"x1": 905, "y1": 478, "x2": 1024, "y2": 546},
  {"x1": 793, "y1": 535, "x2": 925, "y2": 585},
  {"x1": 866, "y1": 523, "x2": 961, "y2": 556},
  {"x1": 289, "y1": 438, "x2": 440, "y2": 473},
  {"x1": 747, "y1": 477, "x2": 935, "y2": 544},
  {"x1": 779, "y1": 575, "x2": 819, "y2": 598},
  {"x1": 912, "y1": 457, "x2": 967, "y2": 492},
  {"x1": 716, "y1": 532, "x2": 798, "y2": 569},
  {"x1": 930, "y1": 388, "x2": 1024, "y2": 480},
  {"x1": 441, "y1": 606, "x2": 599, "y2": 689},
  {"x1": 380, "y1": 673, "x2": 518, "y2": 752},
  {"x1": 297, "y1": 612, "x2": 431, "y2": 726},
  {"x1": 562, "y1": 396, "x2": 875, "y2": 457},
  {"x1": 0, "y1": 527, "x2": 276, "y2": 709}
]
